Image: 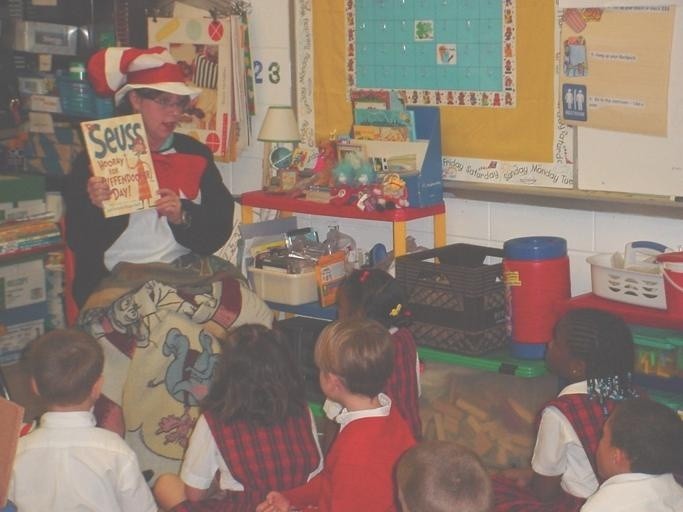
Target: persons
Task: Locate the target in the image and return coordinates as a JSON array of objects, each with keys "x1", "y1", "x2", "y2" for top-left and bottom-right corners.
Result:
[{"x1": 62, "y1": 43, "x2": 273, "y2": 431}]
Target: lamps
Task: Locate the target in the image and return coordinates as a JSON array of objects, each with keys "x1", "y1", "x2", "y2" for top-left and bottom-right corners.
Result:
[{"x1": 256, "y1": 105, "x2": 302, "y2": 185}]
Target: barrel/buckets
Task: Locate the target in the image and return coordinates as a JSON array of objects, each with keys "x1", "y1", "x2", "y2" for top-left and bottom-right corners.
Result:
[
  {"x1": 503, "y1": 236, "x2": 571, "y2": 359},
  {"x1": 657, "y1": 252, "x2": 683, "y2": 312}
]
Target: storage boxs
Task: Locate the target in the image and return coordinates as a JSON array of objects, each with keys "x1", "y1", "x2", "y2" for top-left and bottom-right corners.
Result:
[
  {"x1": 246, "y1": 263, "x2": 320, "y2": 305},
  {"x1": 2, "y1": 3, "x2": 95, "y2": 179},
  {"x1": 414, "y1": 344, "x2": 558, "y2": 478},
  {"x1": 2, "y1": 252, "x2": 54, "y2": 370},
  {"x1": 629, "y1": 329, "x2": 681, "y2": 381}
]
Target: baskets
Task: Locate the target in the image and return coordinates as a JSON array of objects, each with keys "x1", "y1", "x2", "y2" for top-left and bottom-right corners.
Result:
[{"x1": 583, "y1": 245, "x2": 668, "y2": 311}]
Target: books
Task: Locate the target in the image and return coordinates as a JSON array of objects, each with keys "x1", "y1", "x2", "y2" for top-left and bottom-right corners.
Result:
[{"x1": 80, "y1": 114, "x2": 162, "y2": 220}]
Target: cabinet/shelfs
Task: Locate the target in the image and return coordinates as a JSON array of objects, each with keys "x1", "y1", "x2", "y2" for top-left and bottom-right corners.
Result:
[{"x1": 234, "y1": 186, "x2": 448, "y2": 322}]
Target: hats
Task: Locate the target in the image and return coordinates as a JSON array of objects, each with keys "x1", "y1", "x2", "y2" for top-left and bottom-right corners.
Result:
[{"x1": 82, "y1": 45, "x2": 203, "y2": 106}]
